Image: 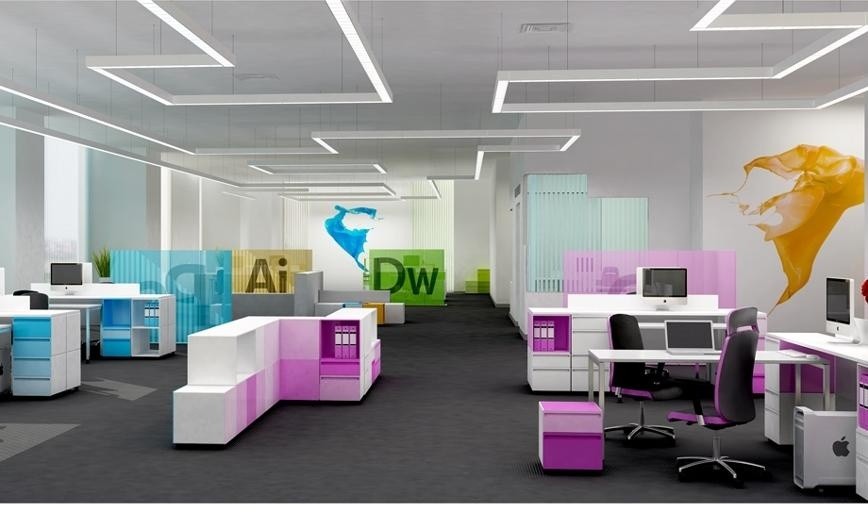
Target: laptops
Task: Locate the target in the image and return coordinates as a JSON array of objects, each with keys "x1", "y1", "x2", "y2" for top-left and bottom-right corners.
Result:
[{"x1": 664, "y1": 320, "x2": 722, "y2": 358}]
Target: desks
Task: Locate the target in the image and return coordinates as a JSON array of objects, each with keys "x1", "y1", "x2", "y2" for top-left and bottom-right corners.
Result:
[
  {"x1": 764, "y1": 331, "x2": 866, "y2": 500},
  {"x1": 526, "y1": 291, "x2": 769, "y2": 395},
  {"x1": 587, "y1": 348, "x2": 829, "y2": 445},
  {"x1": 0, "y1": 283, "x2": 177, "y2": 397}
]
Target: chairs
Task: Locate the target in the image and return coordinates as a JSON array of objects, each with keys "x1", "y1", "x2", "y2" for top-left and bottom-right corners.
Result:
[
  {"x1": 668, "y1": 306, "x2": 766, "y2": 482},
  {"x1": 599, "y1": 313, "x2": 676, "y2": 439}
]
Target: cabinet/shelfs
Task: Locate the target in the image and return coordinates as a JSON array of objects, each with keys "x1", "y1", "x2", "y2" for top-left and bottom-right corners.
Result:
[
  {"x1": 173, "y1": 308, "x2": 381, "y2": 444},
  {"x1": 539, "y1": 400, "x2": 604, "y2": 472}
]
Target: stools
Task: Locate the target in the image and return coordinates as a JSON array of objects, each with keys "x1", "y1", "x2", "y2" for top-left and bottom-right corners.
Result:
[{"x1": 314, "y1": 303, "x2": 405, "y2": 325}]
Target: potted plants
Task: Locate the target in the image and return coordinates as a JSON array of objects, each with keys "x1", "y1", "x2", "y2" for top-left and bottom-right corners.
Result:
[{"x1": 90, "y1": 248, "x2": 111, "y2": 283}]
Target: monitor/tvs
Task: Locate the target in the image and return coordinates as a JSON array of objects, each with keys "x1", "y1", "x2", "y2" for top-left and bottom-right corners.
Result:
[
  {"x1": 50, "y1": 262, "x2": 84, "y2": 296},
  {"x1": 825, "y1": 277, "x2": 862, "y2": 345},
  {"x1": 643, "y1": 268, "x2": 687, "y2": 311}
]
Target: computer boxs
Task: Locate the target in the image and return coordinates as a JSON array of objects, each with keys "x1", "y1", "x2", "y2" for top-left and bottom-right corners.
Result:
[
  {"x1": 0, "y1": 324, "x2": 11, "y2": 398},
  {"x1": 793, "y1": 405, "x2": 856, "y2": 494}
]
